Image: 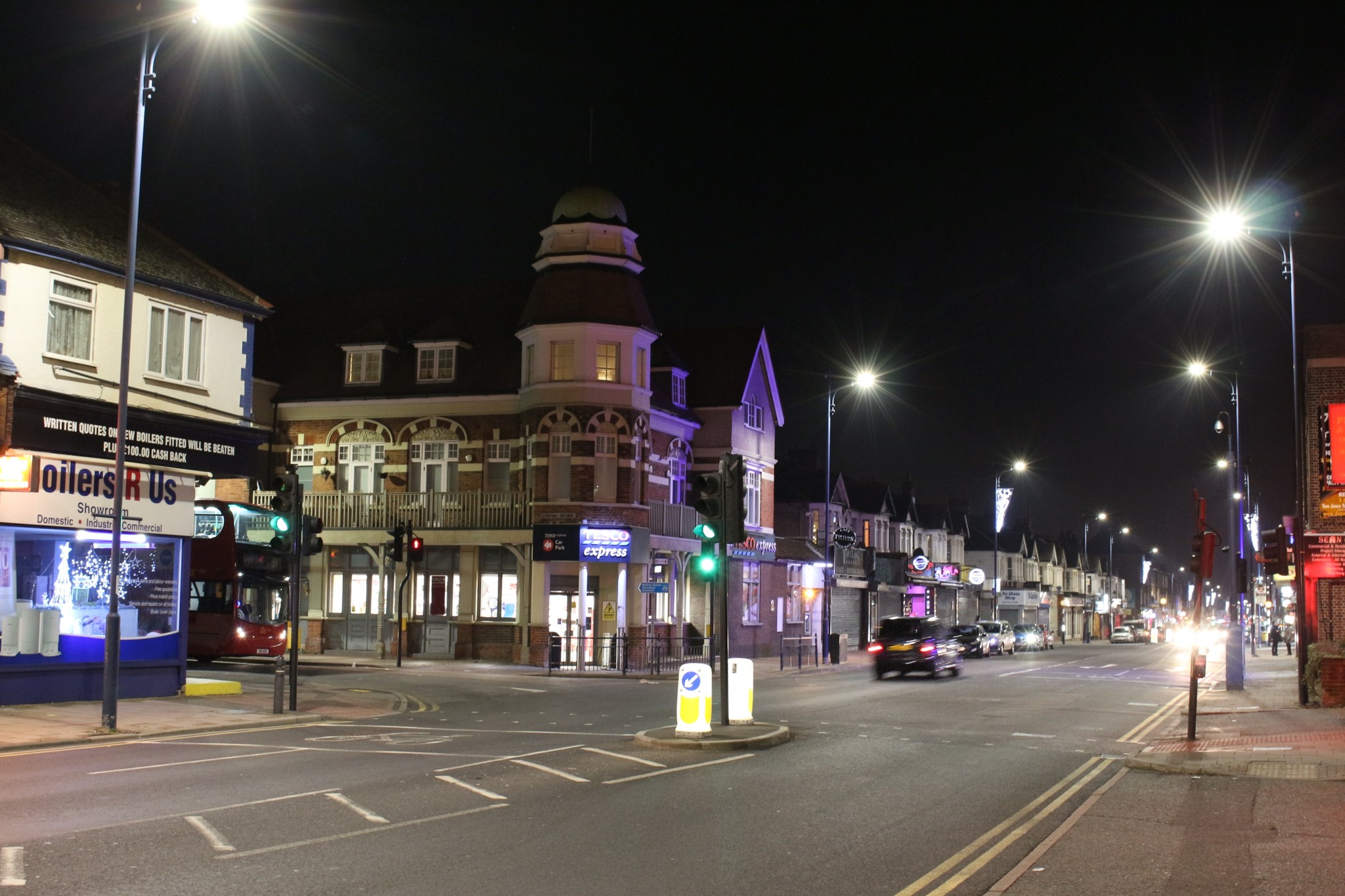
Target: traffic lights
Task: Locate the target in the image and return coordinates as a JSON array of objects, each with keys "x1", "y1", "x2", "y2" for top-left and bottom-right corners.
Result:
[
  {"x1": 271, "y1": 474, "x2": 297, "y2": 553},
  {"x1": 1260, "y1": 525, "x2": 1290, "y2": 577},
  {"x1": 720, "y1": 454, "x2": 749, "y2": 543},
  {"x1": 383, "y1": 527, "x2": 404, "y2": 563},
  {"x1": 411, "y1": 538, "x2": 425, "y2": 561},
  {"x1": 692, "y1": 473, "x2": 724, "y2": 544},
  {"x1": 1190, "y1": 532, "x2": 1214, "y2": 578},
  {"x1": 303, "y1": 513, "x2": 324, "y2": 556},
  {"x1": 694, "y1": 542, "x2": 716, "y2": 582}
]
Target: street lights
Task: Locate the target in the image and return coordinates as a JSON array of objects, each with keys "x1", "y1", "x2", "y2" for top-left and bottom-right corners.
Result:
[
  {"x1": 993, "y1": 460, "x2": 1029, "y2": 621},
  {"x1": 100, "y1": 0, "x2": 268, "y2": 732},
  {"x1": 1083, "y1": 512, "x2": 1106, "y2": 644},
  {"x1": 1178, "y1": 170, "x2": 1309, "y2": 710},
  {"x1": 1109, "y1": 527, "x2": 1129, "y2": 639},
  {"x1": 821, "y1": 366, "x2": 879, "y2": 660}
]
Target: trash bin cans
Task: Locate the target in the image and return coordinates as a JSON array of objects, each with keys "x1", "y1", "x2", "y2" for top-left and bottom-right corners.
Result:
[
  {"x1": 831, "y1": 632, "x2": 848, "y2": 665},
  {"x1": 550, "y1": 631, "x2": 562, "y2": 667}
]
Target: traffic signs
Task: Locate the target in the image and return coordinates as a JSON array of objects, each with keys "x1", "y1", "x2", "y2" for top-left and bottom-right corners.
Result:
[
  {"x1": 655, "y1": 558, "x2": 670, "y2": 565},
  {"x1": 534, "y1": 525, "x2": 581, "y2": 561},
  {"x1": 638, "y1": 582, "x2": 669, "y2": 594}
]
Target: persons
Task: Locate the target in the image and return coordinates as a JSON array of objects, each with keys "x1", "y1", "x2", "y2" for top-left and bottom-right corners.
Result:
[
  {"x1": 1270, "y1": 621, "x2": 1280, "y2": 656},
  {"x1": 237, "y1": 604, "x2": 252, "y2": 622},
  {"x1": 1284, "y1": 623, "x2": 1292, "y2": 655}
]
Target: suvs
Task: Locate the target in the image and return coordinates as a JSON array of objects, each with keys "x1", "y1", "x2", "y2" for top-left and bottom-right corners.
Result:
[{"x1": 874, "y1": 614, "x2": 963, "y2": 681}]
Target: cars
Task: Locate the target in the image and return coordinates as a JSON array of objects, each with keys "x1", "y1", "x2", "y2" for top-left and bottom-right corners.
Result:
[
  {"x1": 1011, "y1": 624, "x2": 1045, "y2": 652},
  {"x1": 946, "y1": 624, "x2": 991, "y2": 658},
  {"x1": 1037, "y1": 623, "x2": 1055, "y2": 650},
  {"x1": 1110, "y1": 627, "x2": 1134, "y2": 644},
  {"x1": 977, "y1": 620, "x2": 1015, "y2": 655}
]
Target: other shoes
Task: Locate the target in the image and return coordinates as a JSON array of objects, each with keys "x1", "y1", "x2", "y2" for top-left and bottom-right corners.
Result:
[
  {"x1": 1287, "y1": 653, "x2": 1291, "y2": 655},
  {"x1": 1273, "y1": 654, "x2": 1278, "y2": 655}
]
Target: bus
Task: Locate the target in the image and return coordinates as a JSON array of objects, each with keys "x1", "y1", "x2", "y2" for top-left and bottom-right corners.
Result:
[{"x1": 183, "y1": 499, "x2": 289, "y2": 666}]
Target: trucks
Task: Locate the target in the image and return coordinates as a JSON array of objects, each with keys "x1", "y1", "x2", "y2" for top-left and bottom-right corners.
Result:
[{"x1": 1123, "y1": 619, "x2": 1151, "y2": 642}]
